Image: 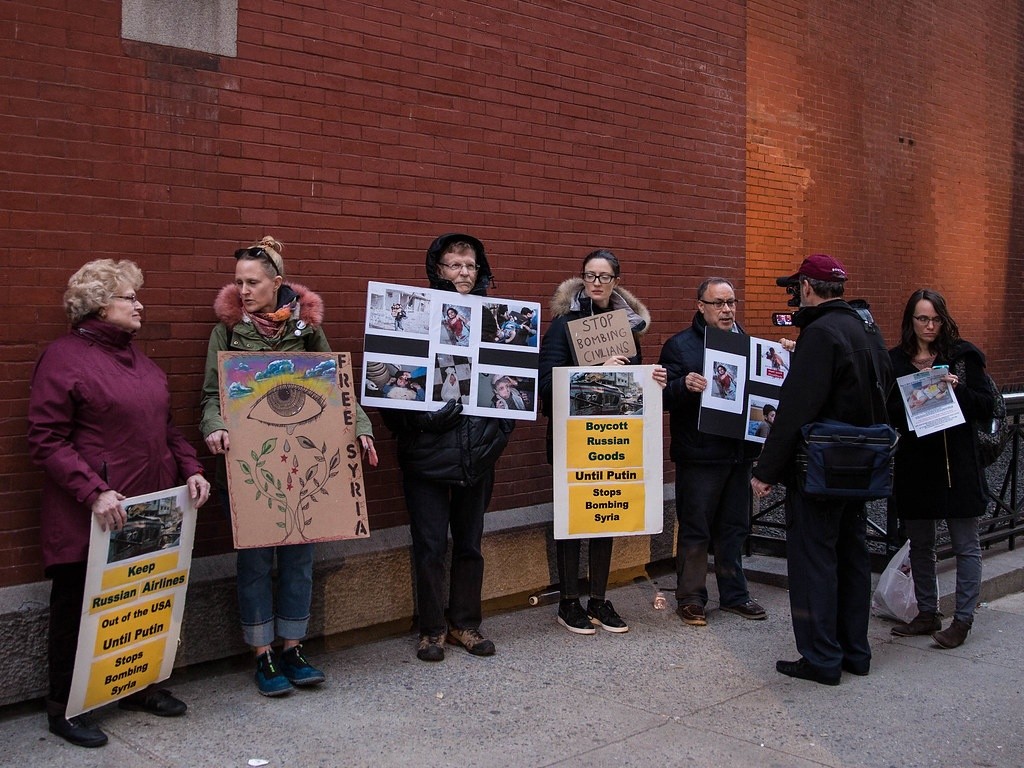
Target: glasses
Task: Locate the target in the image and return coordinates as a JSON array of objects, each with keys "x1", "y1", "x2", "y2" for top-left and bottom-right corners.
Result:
[
  {"x1": 581, "y1": 270, "x2": 617, "y2": 284},
  {"x1": 234, "y1": 247, "x2": 279, "y2": 274},
  {"x1": 913, "y1": 315, "x2": 945, "y2": 326},
  {"x1": 108, "y1": 293, "x2": 137, "y2": 304},
  {"x1": 437, "y1": 261, "x2": 480, "y2": 272},
  {"x1": 700, "y1": 299, "x2": 739, "y2": 310}
]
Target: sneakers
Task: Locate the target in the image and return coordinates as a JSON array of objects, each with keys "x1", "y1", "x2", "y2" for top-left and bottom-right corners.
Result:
[
  {"x1": 446, "y1": 624, "x2": 495, "y2": 656},
  {"x1": 417, "y1": 629, "x2": 448, "y2": 662},
  {"x1": 586, "y1": 597, "x2": 629, "y2": 632},
  {"x1": 48, "y1": 712, "x2": 108, "y2": 747},
  {"x1": 279, "y1": 642, "x2": 325, "y2": 686},
  {"x1": 555, "y1": 603, "x2": 595, "y2": 634},
  {"x1": 253, "y1": 649, "x2": 293, "y2": 696},
  {"x1": 118, "y1": 686, "x2": 187, "y2": 717}
]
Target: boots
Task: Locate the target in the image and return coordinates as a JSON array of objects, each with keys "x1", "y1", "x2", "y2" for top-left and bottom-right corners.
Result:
[
  {"x1": 931, "y1": 615, "x2": 975, "y2": 649},
  {"x1": 891, "y1": 610, "x2": 942, "y2": 636}
]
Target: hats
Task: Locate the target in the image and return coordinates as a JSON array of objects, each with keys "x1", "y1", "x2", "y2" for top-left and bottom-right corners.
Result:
[{"x1": 787, "y1": 254, "x2": 848, "y2": 283}]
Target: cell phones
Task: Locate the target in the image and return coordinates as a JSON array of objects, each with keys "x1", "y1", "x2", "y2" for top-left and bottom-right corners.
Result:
[{"x1": 931, "y1": 366, "x2": 950, "y2": 371}]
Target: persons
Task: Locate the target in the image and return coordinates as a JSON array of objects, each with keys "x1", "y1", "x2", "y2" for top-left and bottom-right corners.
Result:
[
  {"x1": 755, "y1": 404, "x2": 776, "y2": 438},
  {"x1": 909, "y1": 381, "x2": 947, "y2": 410},
  {"x1": 391, "y1": 302, "x2": 406, "y2": 331},
  {"x1": 482, "y1": 304, "x2": 538, "y2": 347},
  {"x1": 658, "y1": 278, "x2": 796, "y2": 626},
  {"x1": 28, "y1": 259, "x2": 210, "y2": 747},
  {"x1": 750, "y1": 255, "x2": 898, "y2": 685},
  {"x1": 381, "y1": 232, "x2": 515, "y2": 661},
  {"x1": 491, "y1": 377, "x2": 534, "y2": 411},
  {"x1": 778, "y1": 315, "x2": 792, "y2": 324},
  {"x1": 365, "y1": 362, "x2": 426, "y2": 400},
  {"x1": 439, "y1": 308, "x2": 467, "y2": 401},
  {"x1": 713, "y1": 365, "x2": 736, "y2": 397},
  {"x1": 887, "y1": 289, "x2": 991, "y2": 649},
  {"x1": 766, "y1": 347, "x2": 789, "y2": 372},
  {"x1": 199, "y1": 236, "x2": 378, "y2": 696},
  {"x1": 125, "y1": 503, "x2": 184, "y2": 554},
  {"x1": 537, "y1": 250, "x2": 668, "y2": 634}
]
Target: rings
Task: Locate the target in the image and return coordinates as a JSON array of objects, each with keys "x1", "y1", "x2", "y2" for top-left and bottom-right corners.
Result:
[
  {"x1": 952, "y1": 379, "x2": 956, "y2": 384},
  {"x1": 758, "y1": 492, "x2": 762, "y2": 496}
]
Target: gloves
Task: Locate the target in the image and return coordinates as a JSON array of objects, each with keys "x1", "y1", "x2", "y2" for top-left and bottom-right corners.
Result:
[{"x1": 415, "y1": 399, "x2": 462, "y2": 432}]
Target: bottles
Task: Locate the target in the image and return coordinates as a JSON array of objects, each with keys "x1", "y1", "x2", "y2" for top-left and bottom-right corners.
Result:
[
  {"x1": 529, "y1": 589, "x2": 561, "y2": 606},
  {"x1": 654, "y1": 591, "x2": 667, "y2": 609}
]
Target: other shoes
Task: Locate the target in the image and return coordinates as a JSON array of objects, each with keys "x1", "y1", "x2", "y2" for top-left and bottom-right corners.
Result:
[
  {"x1": 718, "y1": 598, "x2": 767, "y2": 620},
  {"x1": 681, "y1": 603, "x2": 707, "y2": 624},
  {"x1": 841, "y1": 660, "x2": 868, "y2": 677},
  {"x1": 775, "y1": 656, "x2": 840, "y2": 685}
]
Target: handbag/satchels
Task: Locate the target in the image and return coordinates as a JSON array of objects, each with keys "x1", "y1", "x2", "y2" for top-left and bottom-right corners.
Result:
[
  {"x1": 802, "y1": 420, "x2": 900, "y2": 501},
  {"x1": 871, "y1": 538, "x2": 940, "y2": 622}
]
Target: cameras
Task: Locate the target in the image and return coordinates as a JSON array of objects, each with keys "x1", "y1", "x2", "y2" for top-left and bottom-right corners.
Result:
[{"x1": 773, "y1": 311, "x2": 798, "y2": 327}]
